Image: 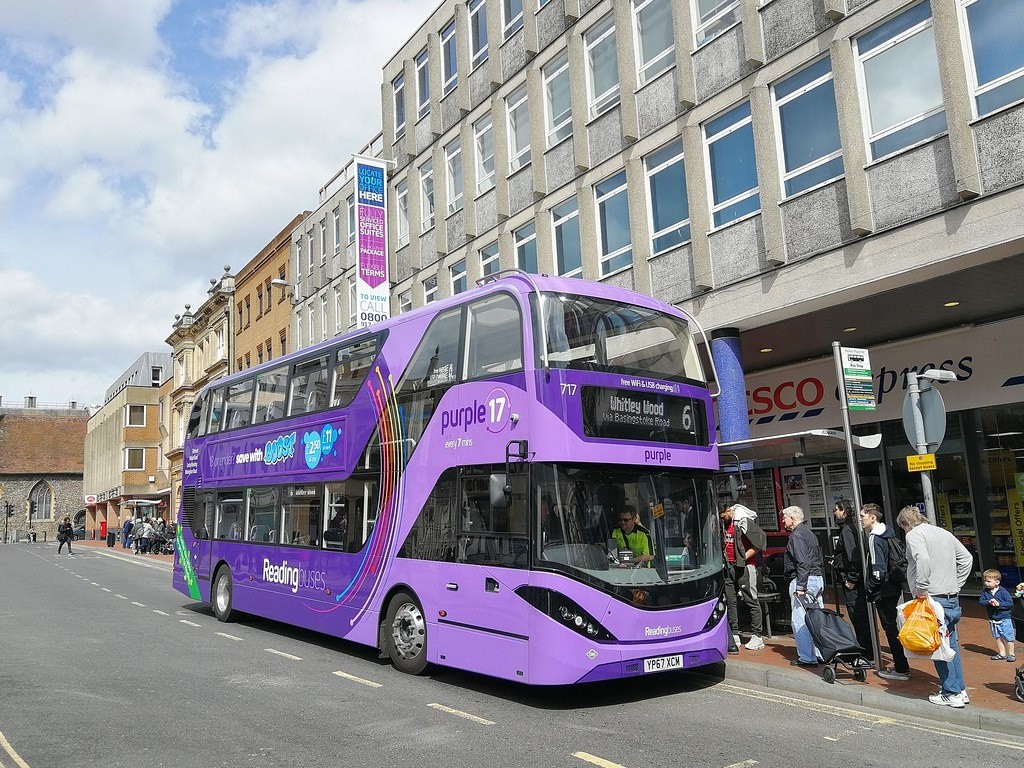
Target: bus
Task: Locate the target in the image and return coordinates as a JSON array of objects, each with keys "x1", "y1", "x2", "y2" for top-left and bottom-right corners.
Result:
[{"x1": 172, "y1": 267, "x2": 747, "y2": 686}]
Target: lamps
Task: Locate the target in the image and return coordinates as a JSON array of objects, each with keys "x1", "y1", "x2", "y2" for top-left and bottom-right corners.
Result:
[{"x1": 272, "y1": 279, "x2": 298, "y2": 303}]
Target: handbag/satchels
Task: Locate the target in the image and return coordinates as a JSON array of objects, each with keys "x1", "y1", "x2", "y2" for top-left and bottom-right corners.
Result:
[
  {"x1": 895, "y1": 594, "x2": 955, "y2": 662},
  {"x1": 58, "y1": 534, "x2": 65, "y2": 541}
]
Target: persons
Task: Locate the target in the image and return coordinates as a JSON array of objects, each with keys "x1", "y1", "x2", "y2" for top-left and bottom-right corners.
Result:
[
  {"x1": 611, "y1": 505, "x2": 657, "y2": 569},
  {"x1": 541, "y1": 498, "x2": 550, "y2": 542},
  {"x1": 782, "y1": 505, "x2": 825, "y2": 668},
  {"x1": 828, "y1": 498, "x2": 875, "y2": 669},
  {"x1": 670, "y1": 495, "x2": 739, "y2": 655},
  {"x1": 717, "y1": 501, "x2": 767, "y2": 651},
  {"x1": 57, "y1": 517, "x2": 75, "y2": 555},
  {"x1": 978, "y1": 568, "x2": 1017, "y2": 662},
  {"x1": 681, "y1": 528, "x2": 697, "y2": 571},
  {"x1": 897, "y1": 506, "x2": 973, "y2": 707},
  {"x1": 123, "y1": 516, "x2": 177, "y2": 555},
  {"x1": 859, "y1": 503, "x2": 912, "y2": 680},
  {"x1": 27, "y1": 526, "x2": 35, "y2": 545}
]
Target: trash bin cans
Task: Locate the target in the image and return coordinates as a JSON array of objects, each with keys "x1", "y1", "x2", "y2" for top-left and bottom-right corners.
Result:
[
  {"x1": 32, "y1": 532, "x2": 36, "y2": 542},
  {"x1": 107, "y1": 533, "x2": 115, "y2": 547}
]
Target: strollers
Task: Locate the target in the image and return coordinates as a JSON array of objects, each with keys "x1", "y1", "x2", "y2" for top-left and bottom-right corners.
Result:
[
  {"x1": 152, "y1": 534, "x2": 175, "y2": 555},
  {"x1": 1009, "y1": 596, "x2": 1024, "y2": 703}
]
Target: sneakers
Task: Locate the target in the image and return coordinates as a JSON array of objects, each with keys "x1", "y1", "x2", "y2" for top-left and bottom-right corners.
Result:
[
  {"x1": 745, "y1": 635, "x2": 765, "y2": 650},
  {"x1": 929, "y1": 693, "x2": 965, "y2": 707},
  {"x1": 938, "y1": 688, "x2": 969, "y2": 702},
  {"x1": 732, "y1": 635, "x2": 742, "y2": 647},
  {"x1": 878, "y1": 669, "x2": 912, "y2": 680}
]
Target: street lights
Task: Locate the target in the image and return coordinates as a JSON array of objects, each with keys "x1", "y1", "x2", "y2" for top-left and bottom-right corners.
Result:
[{"x1": 907, "y1": 370, "x2": 956, "y2": 527}]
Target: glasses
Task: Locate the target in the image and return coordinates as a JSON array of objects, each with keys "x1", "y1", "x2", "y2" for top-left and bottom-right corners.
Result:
[{"x1": 617, "y1": 516, "x2": 635, "y2": 523}]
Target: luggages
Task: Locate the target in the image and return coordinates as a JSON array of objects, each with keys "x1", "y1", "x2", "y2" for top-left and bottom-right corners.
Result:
[{"x1": 794, "y1": 591, "x2": 866, "y2": 683}]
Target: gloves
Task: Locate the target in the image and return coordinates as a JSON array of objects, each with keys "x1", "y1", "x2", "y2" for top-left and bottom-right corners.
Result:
[{"x1": 867, "y1": 580, "x2": 881, "y2": 601}]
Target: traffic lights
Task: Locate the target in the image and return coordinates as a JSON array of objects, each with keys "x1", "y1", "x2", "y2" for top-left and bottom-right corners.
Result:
[
  {"x1": 9, "y1": 505, "x2": 15, "y2": 517},
  {"x1": 31, "y1": 501, "x2": 37, "y2": 514}
]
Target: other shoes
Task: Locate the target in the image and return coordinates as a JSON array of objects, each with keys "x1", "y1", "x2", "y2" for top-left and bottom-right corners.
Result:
[
  {"x1": 68, "y1": 552, "x2": 75, "y2": 555},
  {"x1": 1007, "y1": 654, "x2": 1016, "y2": 661},
  {"x1": 854, "y1": 658, "x2": 876, "y2": 669},
  {"x1": 991, "y1": 654, "x2": 1006, "y2": 660},
  {"x1": 790, "y1": 659, "x2": 817, "y2": 667},
  {"x1": 58, "y1": 553, "x2": 62, "y2": 556}
]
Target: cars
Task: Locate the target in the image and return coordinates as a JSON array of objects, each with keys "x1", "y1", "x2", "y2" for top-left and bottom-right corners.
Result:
[{"x1": 57, "y1": 524, "x2": 85, "y2": 542}]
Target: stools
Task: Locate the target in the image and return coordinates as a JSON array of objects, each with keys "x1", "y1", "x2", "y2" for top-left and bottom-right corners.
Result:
[{"x1": 757, "y1": 592, "x2": 781, "y2": 640}]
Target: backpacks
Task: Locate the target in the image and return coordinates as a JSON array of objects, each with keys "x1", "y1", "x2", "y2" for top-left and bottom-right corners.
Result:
[{"x1": 886, "y1": 537, "x2": 911, "y2": 594}]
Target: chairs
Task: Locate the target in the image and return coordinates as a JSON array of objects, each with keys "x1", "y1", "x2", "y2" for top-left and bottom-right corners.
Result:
[
  {"x1": 228, "y1": 521, "x2": 310, "y2": 546},
  {"x1": 231, "y1": 391, "x2": 329, "y2": 429}
]
